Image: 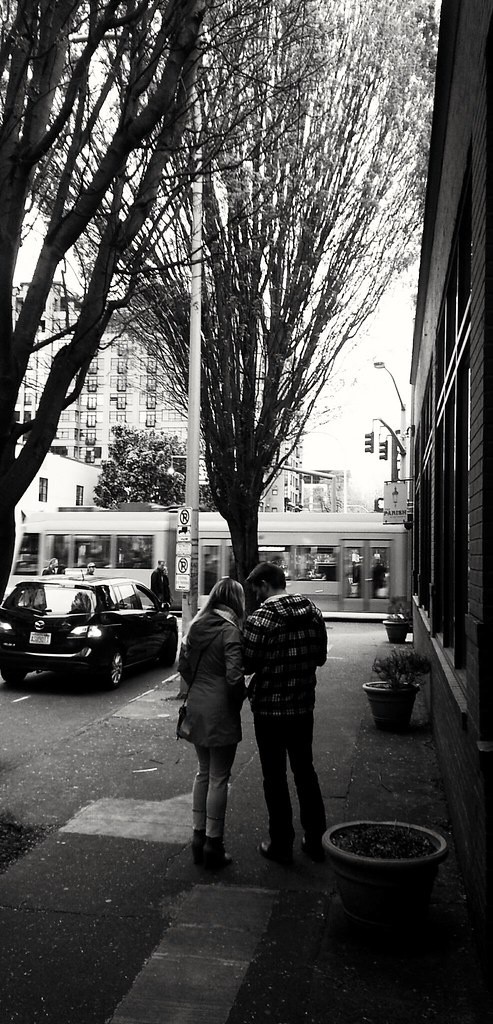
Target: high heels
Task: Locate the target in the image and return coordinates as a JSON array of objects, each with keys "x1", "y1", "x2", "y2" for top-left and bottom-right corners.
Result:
[
  {"x1": 192, "y1": 840, "x2": 205, "y2": 862},
  {"x1": 204, "y1": 845, "x2": 231, "y2": 869}
]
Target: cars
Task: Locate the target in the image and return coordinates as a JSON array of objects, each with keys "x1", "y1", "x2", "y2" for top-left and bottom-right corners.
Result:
[{"x1": 0, "y1": 570, "x2": 178, "y2": 690}]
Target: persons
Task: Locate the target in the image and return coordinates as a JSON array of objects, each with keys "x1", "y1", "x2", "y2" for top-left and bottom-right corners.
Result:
[
  {"x1": 351, "y1": 558, "x2": 390, "y2": 598},
  {"x1": 85, "y1": 562, "x2": 96, "y2": 576},
  {"x1": 41, "y1": 558, "x2": 58, "y2": 576},
  {"x1": 163, "y1": 566, "x2": 175, "y2": 605},
  {"x1": 178, "y1": 576, "x2": 248, "y2": 870},
  {"x1": 151, "y1": 559, "x2": 170, "y2": 612},
  {"x1": 241, "y1": 562, "x2": 328, "y2": 864},
  {"x1": 56, "y1": 564, "x2": 67, "y2": 574}
]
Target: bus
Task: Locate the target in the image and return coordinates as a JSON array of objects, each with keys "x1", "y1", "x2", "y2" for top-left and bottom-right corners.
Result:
[{"x1": 5, "y1": 508, "x2": 408, "y2": 619}]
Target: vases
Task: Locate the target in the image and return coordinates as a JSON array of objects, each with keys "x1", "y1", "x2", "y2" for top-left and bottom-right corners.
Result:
[{"x1": 324, "y1": 819, "x2": 445, "y2": 933}]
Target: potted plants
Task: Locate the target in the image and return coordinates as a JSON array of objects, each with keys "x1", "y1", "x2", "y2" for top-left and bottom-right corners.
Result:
[
  {"x1": 383, "y1": 595, "x2": 409, "y2": 643},
  {"x1": 364, "y1": 644, "x2": 431, "y2": 731}
]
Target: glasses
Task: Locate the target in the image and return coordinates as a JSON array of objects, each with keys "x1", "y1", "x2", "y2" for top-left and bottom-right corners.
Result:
[{"x1": 88, "y1": 566, "x2": 94, "y2": 568}]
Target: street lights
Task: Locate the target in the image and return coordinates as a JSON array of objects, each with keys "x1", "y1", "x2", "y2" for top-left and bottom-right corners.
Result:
[
  {"x1": 374, "y1": 362, "x2": 407, "y2": 483},
  {"x1": 168, "y1": 456, "x2": 337, "y2": 513}
]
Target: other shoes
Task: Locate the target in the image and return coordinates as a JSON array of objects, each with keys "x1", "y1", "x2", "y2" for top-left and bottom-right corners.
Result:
[
  {"x1": 260, "y1": 841, "x2": 271, "y2": 856},
  {"x1": 302, "y1": 837, "x2": 306, "y2": 846}
]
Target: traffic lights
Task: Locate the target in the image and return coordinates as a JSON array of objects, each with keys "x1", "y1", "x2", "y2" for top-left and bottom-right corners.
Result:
[
  {"x1": 379, "y1": 440, "x2": 388, "y2": 460},
  {"x1": 365, "y1": 432, "x2": 374, "y2": 453}
]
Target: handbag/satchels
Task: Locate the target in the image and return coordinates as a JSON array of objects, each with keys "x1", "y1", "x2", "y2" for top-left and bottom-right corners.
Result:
[{"x1": 176, "y1": 706, "x2": 192, "y2": 743}]
